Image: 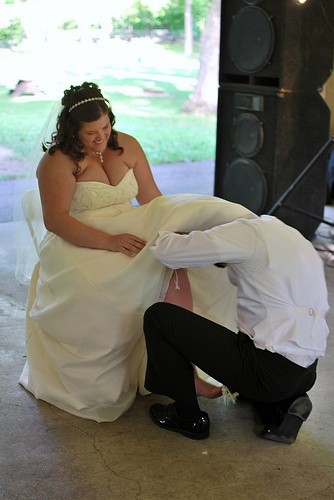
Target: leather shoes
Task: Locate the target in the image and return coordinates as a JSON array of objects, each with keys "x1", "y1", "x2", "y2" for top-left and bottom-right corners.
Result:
[
  {"x1": 261, "y1": 393, "x2": 312, "y2": 444},
  {"x1": 149, "y1": 402, "x2": 210, "y2": 440}
]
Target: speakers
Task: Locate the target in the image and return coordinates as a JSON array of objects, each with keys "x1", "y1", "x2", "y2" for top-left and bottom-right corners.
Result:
[
  {"x1": 218, "y1": 0, "x2": 334, "y2": 92},
  {"x1": 214, "y1": 87, "x2": 330, "y2": 242}
]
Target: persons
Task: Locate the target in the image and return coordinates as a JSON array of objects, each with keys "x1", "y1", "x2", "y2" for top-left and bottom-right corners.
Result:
[
  {"x1": 142, "y1": 213, "x2": 329, "y2": 445},
  {"x1": 15, "y1": 81, "x2": 259, "y2": 424}
]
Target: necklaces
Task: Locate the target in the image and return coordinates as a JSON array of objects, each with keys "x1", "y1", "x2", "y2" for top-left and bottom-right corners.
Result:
[{"x1": 72, "y1": 145, "x2": 117, "y2": 164}]
